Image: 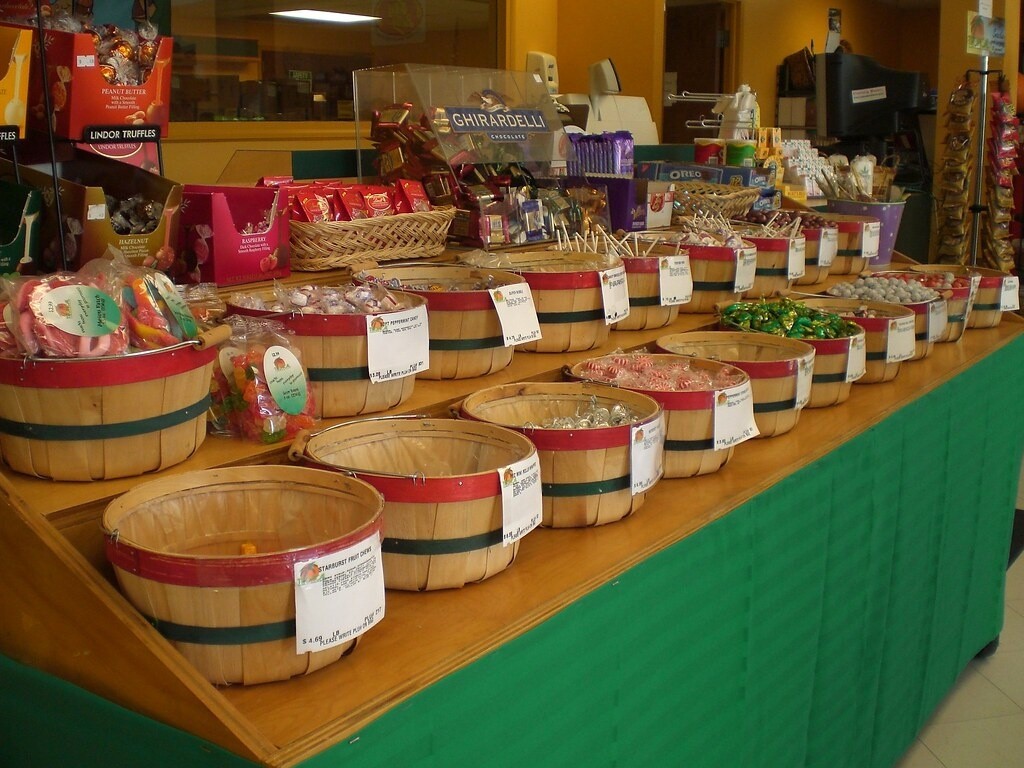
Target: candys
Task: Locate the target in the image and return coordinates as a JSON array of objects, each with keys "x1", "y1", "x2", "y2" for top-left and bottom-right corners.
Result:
[
  {"x1": 633, "y1": 226, "x2": 750, "y2": 248},
  {"x1": 249, "y1": 275, "x2": 512, "y2": 314},
  {"x1": 205, "y1": 344, "x2": 321, "y2": 446},
  {"x1": 68, "y1": 19, "x2": 160, "y2": 88},
  {"x1": 521, "y1": 356, "x2": 738, "y2": 428}
]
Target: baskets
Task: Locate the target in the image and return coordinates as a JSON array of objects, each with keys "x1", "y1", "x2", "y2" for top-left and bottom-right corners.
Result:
[
  {"x1": 286, "y1": 204, "x2": 456, "y2": 272},
  {"x1": 668, "y1": 182, "x2": 762, "y2": 221}
]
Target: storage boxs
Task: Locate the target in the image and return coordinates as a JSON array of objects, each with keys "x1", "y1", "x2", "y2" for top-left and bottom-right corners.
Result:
[
  {"x1": 751, "y1": 190, "x2": 783, "y2": 213},
  {"x1": 71, "y1": 142, "x2": 161, "y2": 177},
  {"x1": 768, "y1": 167, "x2": 785, "y2": 185},
  {"x1": 353, "y1": 62, "x2": 613, "y2": 251},
  {"x1": 174, "y1": 184, "x2": 292, "y2": 289},
  {"x1": 536, "y1": 177, "x2": 675, "y2": 232},
  {"x1": 637, "y1": 160, "x2": 723, "y2": 187},
  {"x1": 0, "y1": 144, "x2": 183, "y2": 271},
  {"x1": 776, "y1": 183, "x2": 809, "y2": 202},
  {"x1": 720, "y1": 168, "x2": 775, "y2": 190},
  {"x1": 0, "y1": 181, "x2": 43, "y2": 275},
  {"x1": 0, "y1": 22, "x2": 173, "y2": 137},
  {"x1": 778, "y1": 94, "x2": 817, "y2": 143},
  {"x1": 0, "y1": 26, "x2": 33, "y2": 141},
  {"x1": 749, "y1": 127, "x2": 786, "y2": 159}
]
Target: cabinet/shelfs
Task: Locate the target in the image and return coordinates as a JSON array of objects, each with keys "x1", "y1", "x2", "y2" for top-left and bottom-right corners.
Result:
[{"x1": 0, "y1": 225, "x2": 1024, "y2": 768}]
